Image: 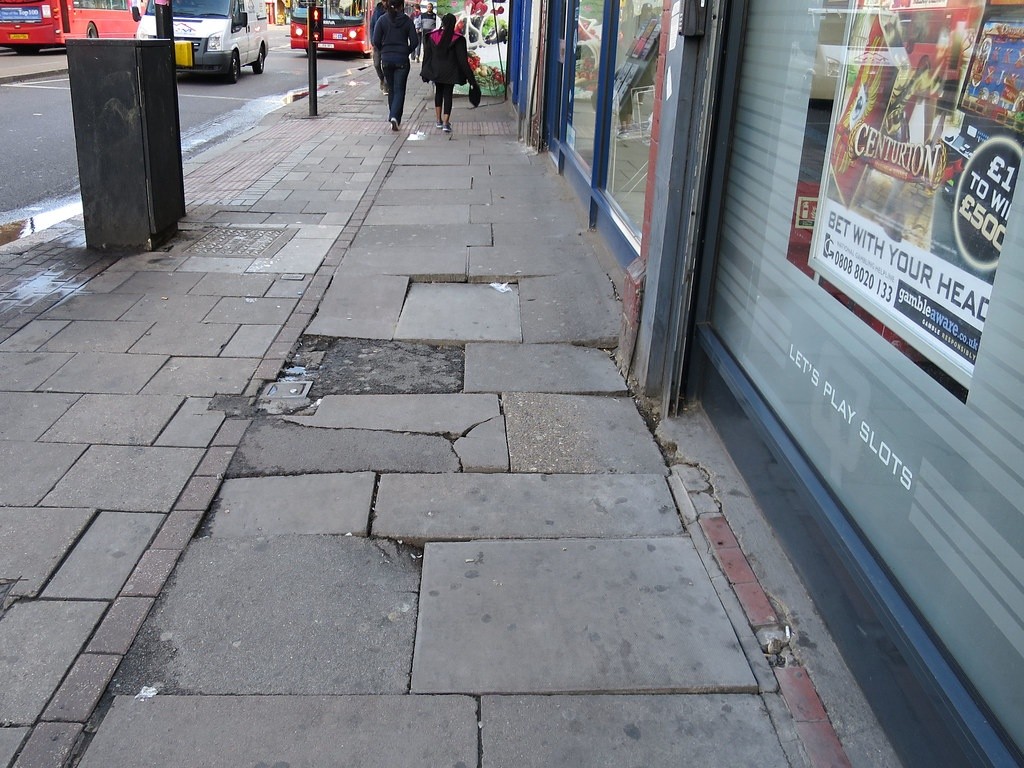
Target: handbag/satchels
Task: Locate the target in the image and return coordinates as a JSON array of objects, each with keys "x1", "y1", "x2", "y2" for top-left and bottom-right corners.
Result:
[{"x1": 468, "y1": 82, "x2": 482, "y2": 108}]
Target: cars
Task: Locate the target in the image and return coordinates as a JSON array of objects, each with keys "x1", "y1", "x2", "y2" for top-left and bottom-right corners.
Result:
[{"x1": 811, "y1": 9, "x2": 910, "y2": 102}]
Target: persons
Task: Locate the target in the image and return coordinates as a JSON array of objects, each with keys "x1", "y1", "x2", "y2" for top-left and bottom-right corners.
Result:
[
  {"x1": 418, "y1": 14, "x2": 478, "y2": 131},
  {"x1": 370, "y1": 0, "x2": 435, "y2": 131}
]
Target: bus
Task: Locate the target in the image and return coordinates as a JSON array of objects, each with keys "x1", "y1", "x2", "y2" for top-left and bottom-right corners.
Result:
[
  {"x1": 894, "y1": 1, "x2": 985, "y2": 81},
  {"x1": 290, "y1": 0, "x2": 376, "y2": 59},
  {"x1": 0, "y1": 1, "x2": 146, "y2": 54}
]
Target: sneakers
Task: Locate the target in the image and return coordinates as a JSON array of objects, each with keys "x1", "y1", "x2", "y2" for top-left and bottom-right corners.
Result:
[
  {"x1": 436, "y1": 121, "x2": 453, "y2": 132},
  {"x1": 617, "y1": 129, "x2": 631, "y2": 139},
  {"x1": 390, "y1": 117, "x2": 401, "y2": 131},
  {"x1": 379, "y1": 79, "x2": 389, "y2": 95}
]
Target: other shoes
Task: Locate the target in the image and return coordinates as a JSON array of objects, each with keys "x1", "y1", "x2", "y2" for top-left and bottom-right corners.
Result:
[{"x1": 412, "y1": 53, "x2": 420, "y2": 64}]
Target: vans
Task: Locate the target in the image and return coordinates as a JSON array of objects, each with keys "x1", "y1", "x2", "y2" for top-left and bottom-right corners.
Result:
[{"x1": 131, "y1": 0, "x2": 269, "y2": 85}]
